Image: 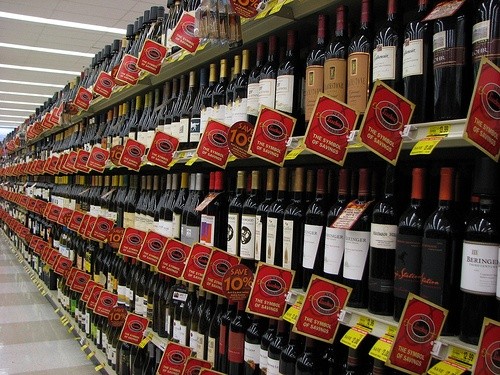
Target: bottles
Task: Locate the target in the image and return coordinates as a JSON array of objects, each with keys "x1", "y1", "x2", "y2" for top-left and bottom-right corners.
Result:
[{"x1": 1, "y1": 1, "x2": 500, "y2": 375}]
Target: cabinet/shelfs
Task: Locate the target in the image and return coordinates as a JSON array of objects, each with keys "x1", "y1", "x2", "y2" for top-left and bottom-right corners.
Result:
[{"x1": 0, "y1": 0, "x2": 500, "y2": 375}]
